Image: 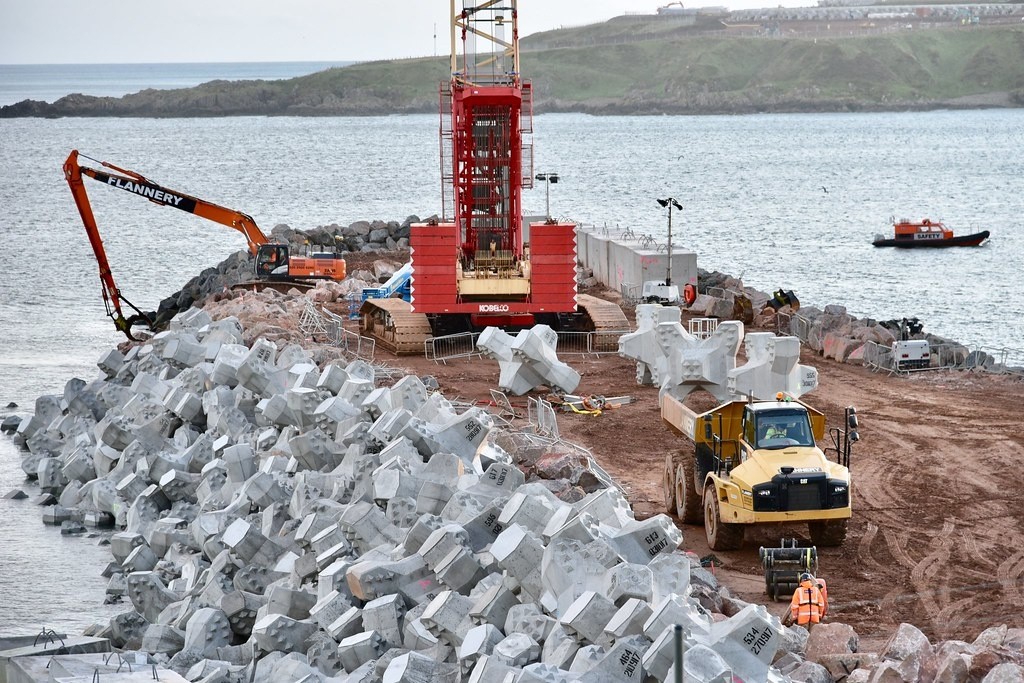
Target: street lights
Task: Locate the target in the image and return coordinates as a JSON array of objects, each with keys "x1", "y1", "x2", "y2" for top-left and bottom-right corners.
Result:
[
  {"x1": 655, "y1": 198, "x2": 683, "y2": 287},
  {"x1": 535, "y1": 172, "x2": 561, "y2": 219}
]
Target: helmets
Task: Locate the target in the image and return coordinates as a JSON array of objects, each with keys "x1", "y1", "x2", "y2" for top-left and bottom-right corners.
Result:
[{"x1": 799, "y1": 572, "x2": 811, "y2": 582}]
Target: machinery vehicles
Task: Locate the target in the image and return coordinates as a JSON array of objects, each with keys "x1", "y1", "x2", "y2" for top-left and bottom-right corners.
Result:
[
  {"x1": 661, "y1": 384, "x2": 859, "y2": 553},
  {"x1": 355, "y1": 0, "x2": 632, "y2": 355},
  {"x1": 61, "y1": 149, "x2": 349, "y2": 344}
]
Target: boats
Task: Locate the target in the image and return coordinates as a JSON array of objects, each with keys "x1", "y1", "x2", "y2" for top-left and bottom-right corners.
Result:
[{"x1": 871, "y1": 217, "x2": 990, "y2": 249}]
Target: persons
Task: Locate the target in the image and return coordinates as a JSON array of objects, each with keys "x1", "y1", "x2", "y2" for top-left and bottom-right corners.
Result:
[
  {"x1": 790, "y1": 573, "x2": 825, "y2": 631},
  {"x1": 764, "y1": 423, "x2": 787, "y2": 440}
]
